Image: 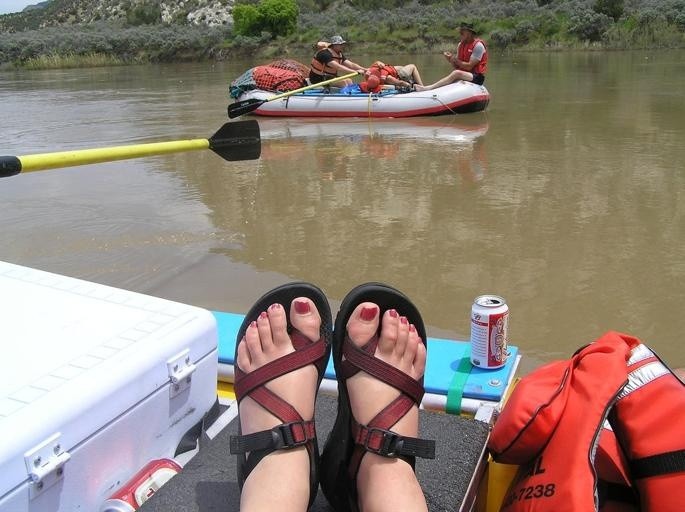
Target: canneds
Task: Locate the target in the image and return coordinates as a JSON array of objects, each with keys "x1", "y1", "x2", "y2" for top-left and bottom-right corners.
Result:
[{"x1": 469, "y1": 295, "x2": 509, "y2": 369}]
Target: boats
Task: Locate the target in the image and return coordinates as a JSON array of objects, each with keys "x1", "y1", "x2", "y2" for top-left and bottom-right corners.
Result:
[{"x1": 235, "y1": 79, "x2": 492, "y2": 119}]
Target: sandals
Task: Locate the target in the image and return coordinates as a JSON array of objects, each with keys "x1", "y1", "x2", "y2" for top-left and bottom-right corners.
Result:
[
  {"x1": 319, "y1": 282, "x2": 436, "y2": 512},
  {"x1": 230, "y1": 281, "x2": 333, "y2": 512}
]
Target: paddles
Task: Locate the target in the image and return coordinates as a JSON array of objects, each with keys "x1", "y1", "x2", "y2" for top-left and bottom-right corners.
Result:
[
  {"x1": 228, "y1": 72, "x2": 361, "y2": 119},
  {"x1": 0, "y1": 119, "x2": 262, "y2": 178}
]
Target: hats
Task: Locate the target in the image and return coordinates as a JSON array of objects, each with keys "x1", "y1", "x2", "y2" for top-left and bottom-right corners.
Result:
[
  {"x1": 455, "y1": 20, "x2": 477, "y2": 34},
  {"x1": 327, "y1": 35, "x2": 348, "y2": 48},
  {"x1": 366, "y1": 73, "x2": 380, "y2": 89}
]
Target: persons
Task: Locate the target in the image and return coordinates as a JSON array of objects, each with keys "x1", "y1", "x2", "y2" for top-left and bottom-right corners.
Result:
[
  {"x1": 231, "y1": 279, "x2": 435, "y2": 511},
  {"x1": 412, "y1": 22, "x2": 487, "y2": 91},
  {"x1": 309, "y1": 34, "x2": 372, "y2": 89},
  {"x1": 364, "y1": 60, "x2": 423, "y2": 90}
]
